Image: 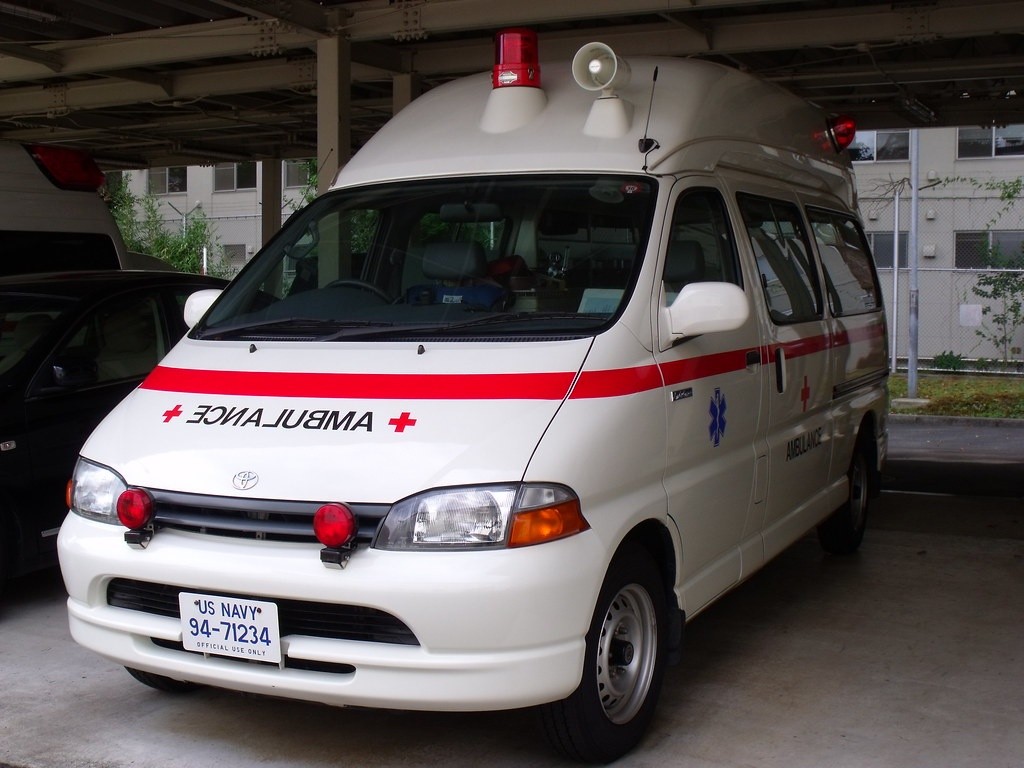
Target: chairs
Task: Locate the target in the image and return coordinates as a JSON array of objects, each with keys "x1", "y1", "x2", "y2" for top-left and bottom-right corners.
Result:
[
  {"x1": 403, "y1": 240, "x2": 508, "y2": 311},
  {"x1": 0, "y1": 314, "x2": 54, "y2": 373},
  {"x1": 95, "y1": 310, "x2": 158, "y2": 382},
  {"x1": 663, "y1": 240, "x2": 706, "y2": 292}
]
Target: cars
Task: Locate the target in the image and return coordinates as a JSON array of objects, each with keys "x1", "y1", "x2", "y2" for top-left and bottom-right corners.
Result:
[{"x1": 0, "y1": 268, "x2": 282, "y2": 600}]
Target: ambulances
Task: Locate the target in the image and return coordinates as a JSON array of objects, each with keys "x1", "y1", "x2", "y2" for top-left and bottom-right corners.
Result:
[{"x1": 56, "y1": 27, "x2": 890, "y2": 767}]
